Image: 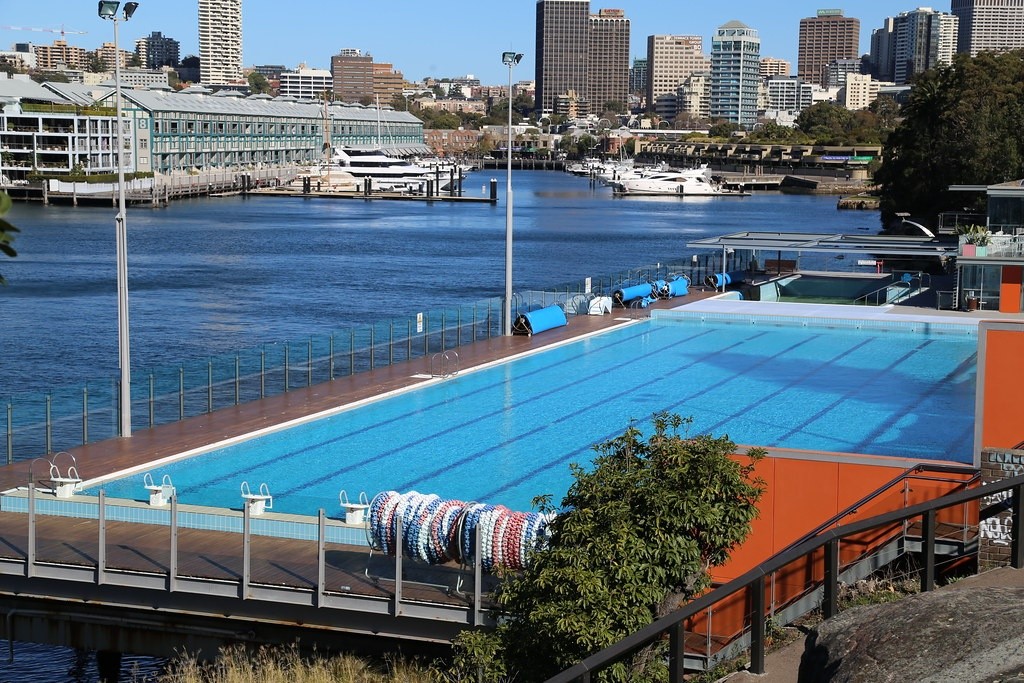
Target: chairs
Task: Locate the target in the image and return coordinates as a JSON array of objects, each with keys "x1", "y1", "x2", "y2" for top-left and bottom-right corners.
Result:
[{"x1": 983, "y1": 227, "x2": 1024, "y2": 257}]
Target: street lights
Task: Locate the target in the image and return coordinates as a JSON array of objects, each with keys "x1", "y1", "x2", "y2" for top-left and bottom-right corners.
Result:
[
  {"x1": 502, "y1": 51, "x2": 524, "y2": 337},
  {"x1": 98, "y1": 1, "x2": 139, "y2": 436}
]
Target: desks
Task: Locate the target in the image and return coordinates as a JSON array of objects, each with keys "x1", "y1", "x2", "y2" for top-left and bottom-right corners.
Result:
[
  {"x1": 936, "y1": 287, "x2": 953, "y2": 310},
  {"x1": 987, "y1": 234, "x2": 1013, "y2": 257}
]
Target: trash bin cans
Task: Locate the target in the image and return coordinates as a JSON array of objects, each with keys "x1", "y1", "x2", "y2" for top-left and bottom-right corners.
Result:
[{"x1": 968, "y1": 297, "x2": 977, "y2": 311}]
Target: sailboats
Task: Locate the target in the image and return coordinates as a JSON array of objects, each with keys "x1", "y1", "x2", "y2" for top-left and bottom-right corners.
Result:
[{"x1": 278, "y1": 89, "x2": 361, "y2": 192}]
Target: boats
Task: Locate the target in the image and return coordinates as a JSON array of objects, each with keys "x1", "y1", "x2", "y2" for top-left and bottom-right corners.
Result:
[
  {"x1": 607, "y1": 174, "x2": 716, "y2": 194},
  {"x1": 568, "y1": 128, "x2": 709, "y2": 186},
  {"x1": 296, "y1": 94, "x2": 473, "y2": 193}
]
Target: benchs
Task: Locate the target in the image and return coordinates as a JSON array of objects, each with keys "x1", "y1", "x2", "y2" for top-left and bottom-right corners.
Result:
[
  {"x1": 765, "y1": 258, "x2": 797, "y2": 272},
  {"x1": 745, "y1": 269, "x2": 767, "y2": 275}
]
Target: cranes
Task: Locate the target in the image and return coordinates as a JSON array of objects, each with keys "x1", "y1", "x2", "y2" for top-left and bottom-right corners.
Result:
[{"x1": 0, "y1": 23, "x2": 88, "y2": 40}]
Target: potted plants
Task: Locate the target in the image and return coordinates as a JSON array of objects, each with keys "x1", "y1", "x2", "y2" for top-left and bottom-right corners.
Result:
[
  {"x1": 954, "y1": 223, "x2": 976, "y2": 257},
  {"x1": 975, "y1": 225, "x2": 993, "y2": 257}
]
